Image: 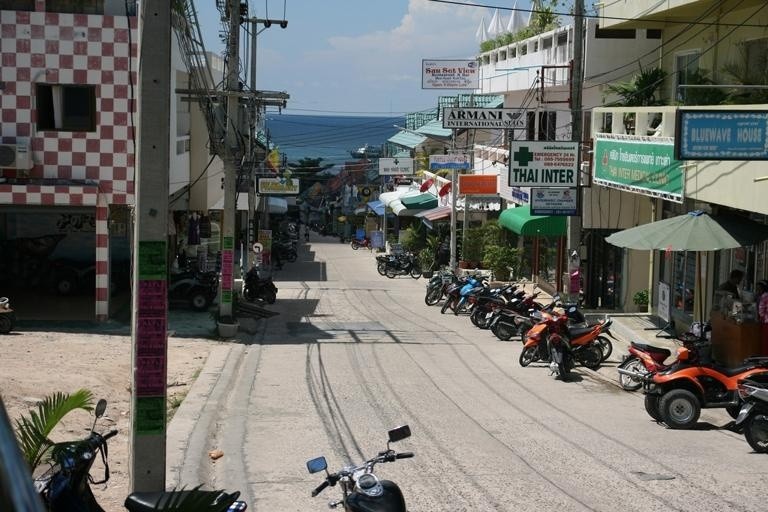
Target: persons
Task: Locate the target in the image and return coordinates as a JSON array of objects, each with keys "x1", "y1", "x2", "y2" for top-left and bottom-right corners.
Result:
[
  {"x1": 754, "y1": 279, "x2": 768, "y2": 325},
  {"x1": 718, "y1": 269, "x2": 745, "y2": 299}
]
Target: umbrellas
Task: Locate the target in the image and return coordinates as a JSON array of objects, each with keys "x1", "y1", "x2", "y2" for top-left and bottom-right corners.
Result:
[{"x1": 603, "y1": 208, "x2": 768, "y2": 341}]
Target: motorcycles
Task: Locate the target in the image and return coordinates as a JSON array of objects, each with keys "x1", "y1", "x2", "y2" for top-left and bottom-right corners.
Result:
[
  {"x1": 243, "y1": 268, "x2": 277, "y2": 304},
  {"x1": 351, "y1": 239, "x2": 373, "y2": 250},
  {"x1": 734, "y1": 378, "x2": 768, "y2": 453},
  {"x1": 168, "y1": 269, "x2": 219, "y2": 311},
  {"x1": 618, "y1": 332, "x2": 707, "y2": 391},
  {"x1": 425, "y1": 270, "x2": 619, "y2": 380},
  {"x1": 628, "y1": 341, "x2": 768, "y2": 430},
  {"x1": 34, "y1": 397, "x2": 247, "y2": 512},
  {"x1": 307, "y1": 425, "x2": 413, "y2": 510},
  {"x1": 377, "y1": 252, "x2": 423, "y2": 279}
]
